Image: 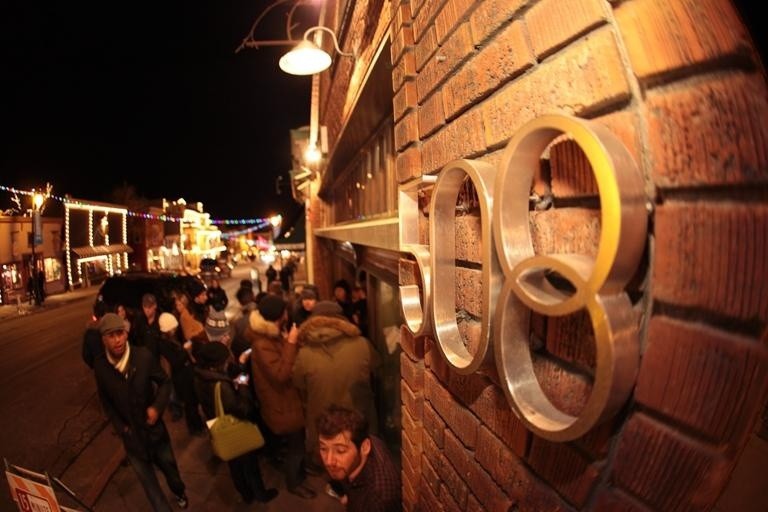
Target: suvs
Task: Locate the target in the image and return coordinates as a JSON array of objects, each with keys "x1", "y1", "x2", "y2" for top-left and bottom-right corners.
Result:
[{"x1": 93, "y1": 271, "x2": 210, "y2": 345}]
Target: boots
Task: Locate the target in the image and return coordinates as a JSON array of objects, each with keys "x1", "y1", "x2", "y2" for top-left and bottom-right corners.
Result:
[{"x1": 167, "y1": 479, "x2": 188, "y2": 508}]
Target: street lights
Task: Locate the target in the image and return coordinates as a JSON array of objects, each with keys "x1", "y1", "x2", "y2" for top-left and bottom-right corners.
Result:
[{"x1": 30, "y1": 194, "x2": 44, "y2": 304}]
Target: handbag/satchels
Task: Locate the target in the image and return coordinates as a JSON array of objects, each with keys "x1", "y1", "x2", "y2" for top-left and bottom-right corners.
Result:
[{"x1": 209, "y1": 381, "x2": 265, "y2": 463}]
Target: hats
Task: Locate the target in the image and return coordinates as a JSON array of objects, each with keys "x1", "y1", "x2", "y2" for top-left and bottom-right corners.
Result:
[
  {"x1": 188, "y1": 283, "x2": 205, "y2": 297},
  {"x1": 141, "y1": 293, "x2": 156, "y2": 306},
  {"x1": 300, "y1": 289, "x2": 345, "y2": 317},
  {"x1": 255, "y1": 292, "x2": 289, "y2": 320},
  {"x1": 158, "y1": 311, "x2": 179, "y2": 333},
  {"x1": 97, "y1": 312, "x2": 124, "y2": 336},
  {"x1": 198, "y1": 340, "x2": 230, "y2": 365}
]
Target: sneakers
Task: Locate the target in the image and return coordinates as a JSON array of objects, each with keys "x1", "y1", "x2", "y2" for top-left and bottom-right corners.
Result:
[
  {"x1": 238, "y1": 489, "x2": 279, "y2": 504},
  {"x1": 288, "y1": 483, "x2": 316, "y2": 499}
]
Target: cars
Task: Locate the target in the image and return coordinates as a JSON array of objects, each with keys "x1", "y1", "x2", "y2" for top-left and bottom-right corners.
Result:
[{"x1": 200, "y1": 259, "x2": 230, "y2": 276}]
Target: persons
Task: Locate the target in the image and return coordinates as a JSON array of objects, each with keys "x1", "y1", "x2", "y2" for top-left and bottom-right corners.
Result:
[
  {"x1": 244, "y1": 295, "x2": 322, "y2": 498},
  {"x1": 116, "y1": 279, "x2": 265, "y2": 433},
  {"x1": 26, "y1": 277, "x2": 39, "y2": 305},
  {"x1": 266, "y1": 261, "x2": 320, "y2": 326},
  {"x1": 193, "y1": 341, "x2": 279, "y2": 503},
  {"x1": 95, "y1": 314, "x2": 189, "y2": 512},
  {"x1": 292, "y1": 301, "x2": 379, "y2": 499},
  {"x1": 317, "y1": 403, "x2": 398, "y2": 512},
  {"x1": 332, "y1": 279, "x2": 368, "y2": 334},
  {"x1": 37, "y1": 268, "x2": 48, "y2": 303}
]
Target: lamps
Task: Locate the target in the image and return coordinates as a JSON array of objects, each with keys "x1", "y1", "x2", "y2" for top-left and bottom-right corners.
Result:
[{"x1": 279, "y1": 25, "x2": 353, "y2": 76}]
